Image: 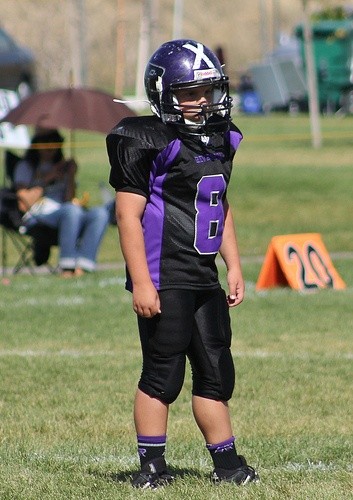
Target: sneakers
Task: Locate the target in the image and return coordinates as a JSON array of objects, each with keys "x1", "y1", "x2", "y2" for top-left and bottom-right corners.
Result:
[
  {"x1": 133, "y1": 472, "x2": 175, "y2": 489},
  {"x1": 212, "y1": 454, "x2": 260, "y2": 487}
]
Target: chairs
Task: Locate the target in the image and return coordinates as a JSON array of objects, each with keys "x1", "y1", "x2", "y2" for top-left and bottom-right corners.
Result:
[{"x1": 0, "y1": 150, "x2": 51, "y2": 279}]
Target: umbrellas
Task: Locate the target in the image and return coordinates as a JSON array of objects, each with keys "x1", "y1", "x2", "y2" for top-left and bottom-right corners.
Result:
[{"x1": 1, "y1": 69, "x2": 138, "y2": 161}]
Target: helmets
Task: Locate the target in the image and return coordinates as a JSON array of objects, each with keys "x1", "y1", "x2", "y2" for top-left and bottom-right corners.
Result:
[{"x1": 144, "y1": 39, "x2": 232, "y2": 136}]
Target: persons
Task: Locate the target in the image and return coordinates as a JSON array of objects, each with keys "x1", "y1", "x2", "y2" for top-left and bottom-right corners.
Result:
[
  {"x1": 106, "y1": 38, "x2": 270, "y2": 488},
  {"x1": 11, "y1": 130, "x2": 111, "y2": 280}
]
